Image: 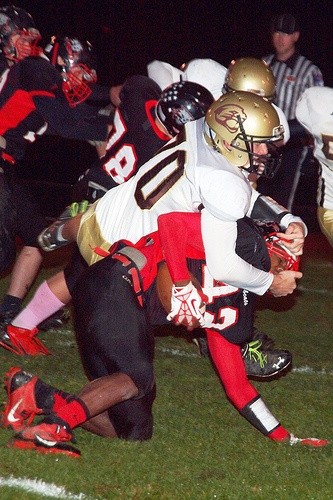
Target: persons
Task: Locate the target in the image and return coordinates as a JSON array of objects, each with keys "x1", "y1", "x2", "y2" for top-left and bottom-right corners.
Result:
[
  {"x1": 296, "y1": 85, "x2": 333, "y2": 247},
  {"x1": 0, "y1": 59, "x2": 307, "y2": 381},
  {"x1": 257, "y1": 15, "x2": 324, "y2": 213},
  {"x1": 0, "y1": 216, "x2": 330, "y2": 457},
  {"x1": 0, "y1": 6, "x2": 34, "y2": 78},
  {"x1": 0, "y1": 32, "x2": 122, "y2": 279}
]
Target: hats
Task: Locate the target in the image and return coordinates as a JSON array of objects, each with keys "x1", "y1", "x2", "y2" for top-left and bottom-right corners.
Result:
[{"x1": 263, "y1": 13, "x2": 299, "y2": 36}]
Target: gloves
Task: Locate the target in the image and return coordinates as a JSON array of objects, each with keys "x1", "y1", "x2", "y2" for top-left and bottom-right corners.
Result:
[
  {"x1": 290, "y1": 432, "x2": 330, "y2": 447},
  {"x1": 165, "y1": 281, "x2": 206, "y2": 331}
]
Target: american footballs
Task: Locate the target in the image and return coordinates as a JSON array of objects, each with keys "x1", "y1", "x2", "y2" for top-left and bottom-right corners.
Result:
[{"x1": 156, "y1": 263, "x2": 206, "y2": 328}]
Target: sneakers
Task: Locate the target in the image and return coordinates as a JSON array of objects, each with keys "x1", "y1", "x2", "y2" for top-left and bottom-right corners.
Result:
[
  {"x1": 0, "y1": 307, "x2": 26, "y2": 330},
  {"x1": 3, "y1": 365, "x2": 44, "y2": 432},
  {"x1": 38, "y1": 309, "x2": 70, "y2": 330},
  {"x1": 238, "y1": 339, "x2": 293, "y2": 377},
  {"x1": 39, "y1": 199, "x2": 100, "y2": 252},
  {"x1": 21, "y1": 415, "x2": 74, "y2": 443},
  {"x1": 1, "y1": 323, "x2": 51, "y2": 358},
  {"x1": 198, "y1": 322, "x2": 275, "y2": 357},
  {"x1": 3, "y1": 424, "x2": 83, "y2": 460}
]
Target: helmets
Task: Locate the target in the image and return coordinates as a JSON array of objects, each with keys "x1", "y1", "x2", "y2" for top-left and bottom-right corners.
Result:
[
  {"x1": 43, "y1": 32, "x2": 101, "y2": 84},
  {"x1": 205, "y1": 91, "x2": 285, "y2": 169},
  {"x1": 222, "y1": 57, "x2": 278, "y2": 105},
  {"x1": 257, "y1": 216, "x2": 301, "y2": 296},
  {"x1": 156, "y1": 74, "x2": 215, "y2": 138},
  {"x1": 0, "y1": 4, "x2": 42, "y2": 69}
]
